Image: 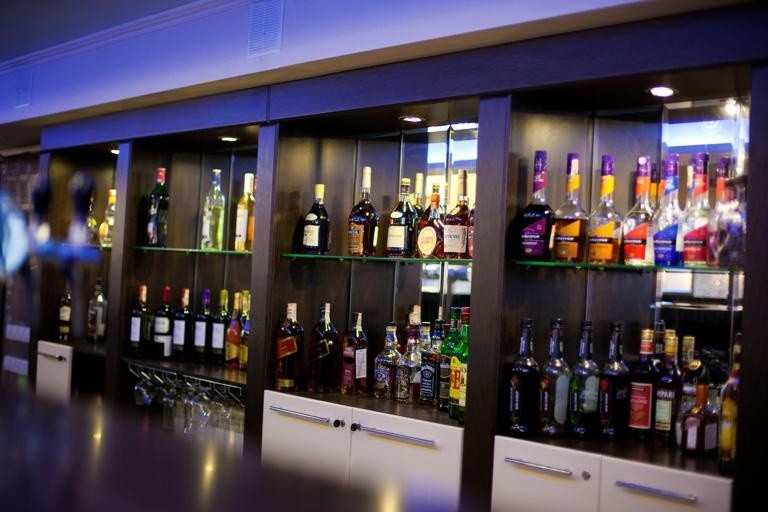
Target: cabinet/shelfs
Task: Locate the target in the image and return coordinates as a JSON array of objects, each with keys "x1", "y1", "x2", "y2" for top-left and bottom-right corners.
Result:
[
  {"x1": 122, "y1": 121, "x2": 258, "y2": 437},
  {"x1": 259, "y1": 98, "x2": 478, "y2": 512},
  {"x1": 34, "y1": 140, "x2": 122, "y2": 408},
  {"x1": 489, "y1": 59, "x2": 768, "y2": 512}
]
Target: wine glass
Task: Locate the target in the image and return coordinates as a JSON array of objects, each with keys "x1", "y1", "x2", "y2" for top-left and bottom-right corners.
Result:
[{"x1": 133, "y1": 376, "x2": 245, "y2": 435}]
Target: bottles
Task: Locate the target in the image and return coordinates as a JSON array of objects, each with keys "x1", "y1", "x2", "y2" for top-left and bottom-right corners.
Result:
[
  {"x1": 508, "y1": 317, "x2": 742, "y2": 477},
  {"x1": 87, "y1": 168, "x2": 256, "y2": 251},
  {"x1": 297, "y1": 167, "x2": 474, "y2": 260},
  {"x1": 273, "y1": 302, "x2": 471, "y2": 426},
  {"x1": 518, "y1": 151, "x2": 749, "y2": 269},
  {"x1": 57, "y1": 279, "x2": 251, "y2": 371}
]
